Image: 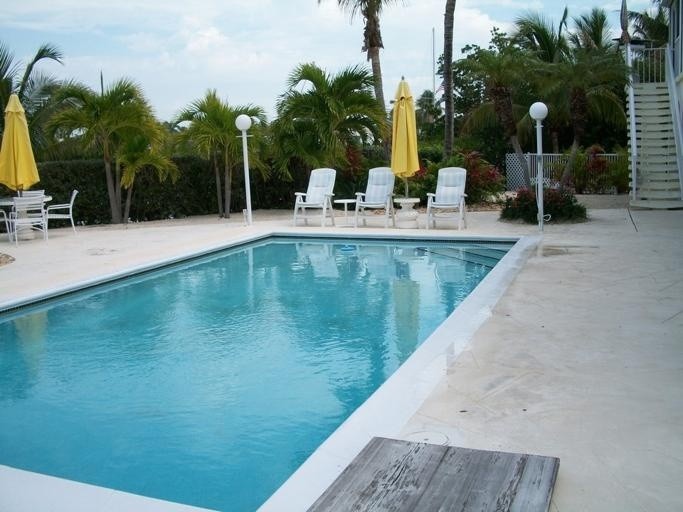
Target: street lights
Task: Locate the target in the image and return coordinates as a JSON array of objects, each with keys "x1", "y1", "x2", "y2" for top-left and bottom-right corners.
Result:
[
  {"x1": 527, "y1": 100, "x2": 549, "y2": 232},
  {"x1": 233, "y1": 112, "x2": 255, "y2": 227}
]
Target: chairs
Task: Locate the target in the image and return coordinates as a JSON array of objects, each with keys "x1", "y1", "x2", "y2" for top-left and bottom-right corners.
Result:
[
  {"x1": 0, "y1": 189, "x2": 52, "y2": 247},
  {"x1": 292, "y1": 166, "x2": 468, "y2": 229},
  {"x1": 45, "y1": 189, "x2": 79, "y2": 242}
]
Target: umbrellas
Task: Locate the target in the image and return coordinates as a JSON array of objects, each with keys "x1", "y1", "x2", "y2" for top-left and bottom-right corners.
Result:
[
  {"x1": 388, "y1": 74, "x2": 421, "y2": 199},
  {"x1": 619, "y1": 0, "x2": 632, "y2": 46},
  {"x1": 0, "y1": 92, "x2": 37, "y2": 196}
]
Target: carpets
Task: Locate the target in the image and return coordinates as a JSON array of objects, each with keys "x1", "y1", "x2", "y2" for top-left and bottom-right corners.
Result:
[{"x1": 303, "y1": 436, "x2": 560, "y2": 512}]
